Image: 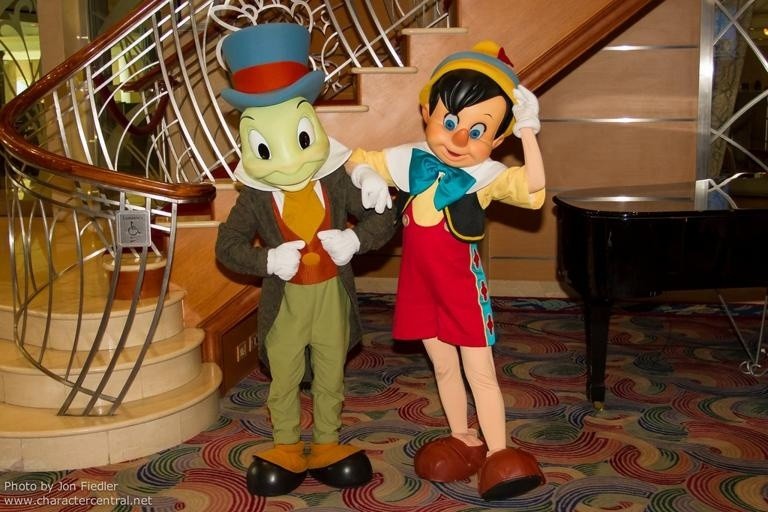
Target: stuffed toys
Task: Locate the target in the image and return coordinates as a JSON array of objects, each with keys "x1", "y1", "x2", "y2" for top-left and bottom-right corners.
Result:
[{"x1": 207, "y1": 19, "x2": 398, "y2": 499}]
